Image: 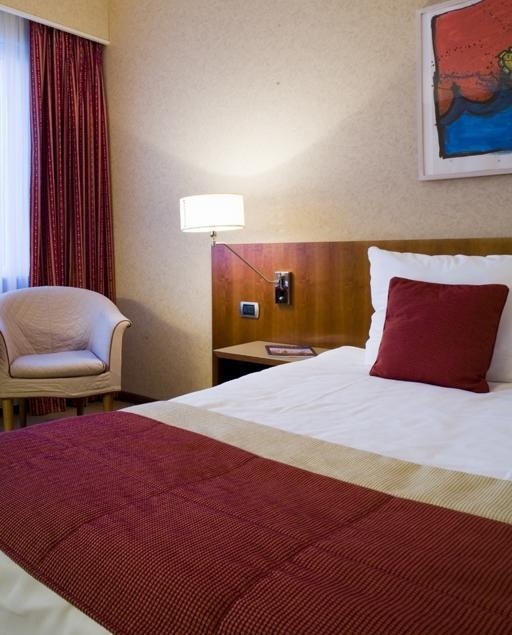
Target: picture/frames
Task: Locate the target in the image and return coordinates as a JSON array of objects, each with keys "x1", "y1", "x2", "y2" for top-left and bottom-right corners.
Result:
[{"x1": 414, "y1": 0, "x2": 512, "y2": 182}]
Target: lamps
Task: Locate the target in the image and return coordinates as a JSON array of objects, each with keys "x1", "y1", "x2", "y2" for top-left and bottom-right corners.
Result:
[{"x1": 179, "y1": 194, "x2": 290, "y2": 304}]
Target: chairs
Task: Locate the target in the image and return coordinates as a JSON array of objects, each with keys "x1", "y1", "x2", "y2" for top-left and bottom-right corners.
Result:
[{"x1": 0, "y1": 286, "x2": 134, "y2": 432}]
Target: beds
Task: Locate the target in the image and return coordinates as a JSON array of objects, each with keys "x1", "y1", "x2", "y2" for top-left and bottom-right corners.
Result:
[{"x1": 0, "y1": 346, "x2": 511, "y2": 635}]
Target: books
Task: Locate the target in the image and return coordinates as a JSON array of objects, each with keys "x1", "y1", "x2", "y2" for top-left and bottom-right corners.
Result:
[{"x1": 265, "y1": 346, "x2": 317, "y2": 361}]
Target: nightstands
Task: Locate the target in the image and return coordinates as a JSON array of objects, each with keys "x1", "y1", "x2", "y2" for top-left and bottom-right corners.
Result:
[{"x1": 213, "y1": 340, "x2": 331, "y2": 387}]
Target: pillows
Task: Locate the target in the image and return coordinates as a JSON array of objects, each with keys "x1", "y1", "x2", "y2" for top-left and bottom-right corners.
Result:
[
  {"x1": 369, "y1": 277, "x2": 509, "y2": 394},
  {"x1": 364, "y1": 246, "x2": 511, "y2": 382}
]
